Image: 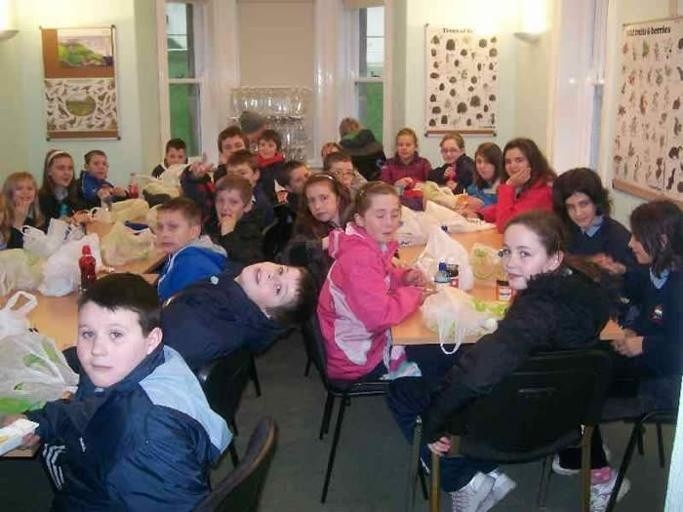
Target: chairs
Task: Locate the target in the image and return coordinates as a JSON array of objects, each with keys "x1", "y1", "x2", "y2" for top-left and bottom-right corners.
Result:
[
  {"x1": 197, "y1": 349, "x2": 250, "y2": 489},
  {"x1": 403, "y1": 349, "x2": 610, "y2": 511},
  {"x1": 301, "y1": 311, "x2": 430, "y2": 502},
  {"x1": 189, "y1": 417, "x2": 279, "y2": 511}
]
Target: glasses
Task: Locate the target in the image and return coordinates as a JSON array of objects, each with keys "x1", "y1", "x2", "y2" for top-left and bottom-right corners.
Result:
[
  {"x1": 330, "y1": 170, "x2": 356, "y2": 180},
  {"x1": 440, "y1": 147, "x2": 463, "y2": 154}
]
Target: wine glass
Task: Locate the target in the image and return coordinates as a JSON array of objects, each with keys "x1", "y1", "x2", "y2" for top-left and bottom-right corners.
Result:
[{"x1": 232, "y1": 88, "x2": 305, "y2": 162}]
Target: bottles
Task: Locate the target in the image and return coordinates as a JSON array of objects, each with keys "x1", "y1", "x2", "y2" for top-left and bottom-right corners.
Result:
[
  {"x1": 439, "y1": 257, "x2": 459, "y2": 288},
  {"x1": 79, "y1": 245, "x2": 97, "y2": 294},
  {"x1": 442, "y1": 226, "x2": 452, "y2": 236},
  {"x1": 101, "y1": 184, "x2": 112, "y2": 212},
  {"x1": 496, "y1": 252, "x2": 513, "y2": 302},
  {"x1": 128, "y1": 173, "x2": 139, "y2": 199},
  {"x1": 434, "y1": 263, "x2": 451, "y2": 293}
]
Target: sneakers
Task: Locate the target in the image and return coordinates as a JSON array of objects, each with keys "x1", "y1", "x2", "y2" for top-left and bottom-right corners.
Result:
[
  {"x1": 590, "y1": 470, "x2": 631, "y2": 511},
  {"x1": 476, "y1": 469, "x2": 516, "y2": 512},
  {"x1": 449, "y1": 471, "x2": 495, "y2": 512}
]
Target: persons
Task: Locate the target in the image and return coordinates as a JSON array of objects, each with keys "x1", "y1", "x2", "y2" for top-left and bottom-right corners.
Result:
[
  {"x1": 159, "y1": 261, "x2": 320, "y2": 373},
  {"x1": 317, "y1": 182, "x2": 434, "y2": 381},
  {"x1": 0, "y1": 273, "x2": 234, "y2": 512},
  {"x1": 0, "y1": 126, "x2": 313, "y2": 300},
  {"x1": 421, "y1": 212, "x2": 611, "y2": 512},
  {"x1": 553, "y1": 197, "x2": 682, "y2": 512},
  {"x1": 300, "y1": 116, "x2": 640, "y2": 278}
]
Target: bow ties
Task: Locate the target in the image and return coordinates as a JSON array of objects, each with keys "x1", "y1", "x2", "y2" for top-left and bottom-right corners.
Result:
[{"x1": 552, "y1": 446, "x2": 610, "y2": 476}]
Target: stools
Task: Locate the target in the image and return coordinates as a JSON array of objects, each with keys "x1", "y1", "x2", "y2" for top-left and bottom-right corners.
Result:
[{"x1": 603, "y1": 407, "x2": 676, "y2": 512}]
